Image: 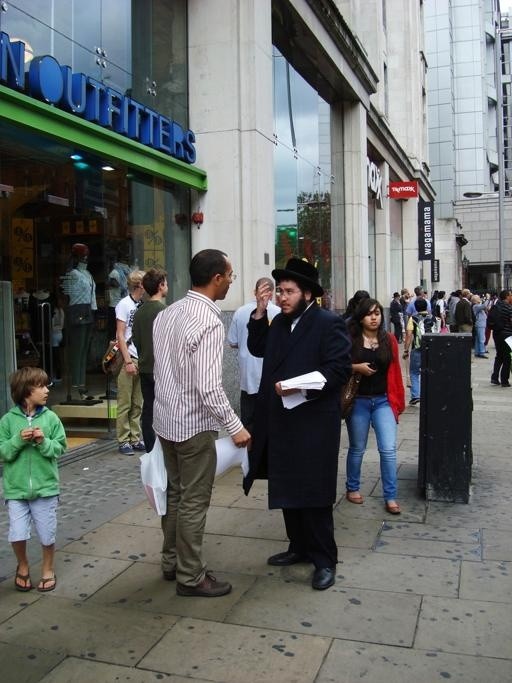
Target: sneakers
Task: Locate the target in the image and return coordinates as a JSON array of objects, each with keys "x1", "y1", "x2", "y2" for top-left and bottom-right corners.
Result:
[
  {"x1": 162, "y1": 566, "x2": 175, "y2": 582},
  {"x1": 409, "y1": 397, "x2": 420, "y2": 404},
  {"x1": 503, "y1": 384, "x2": 512, "y2": 387},
  {"x1": 119, "y1": 443, "x2": 134, "y2": 455},
  {"x1": 175, "y1": 571, "x2": 231, "y2": 597},
  {"x1": 134, "y1": 440, "x2": 148, "y2": 450},
  {"x1": 490, "y1": 379, "x2": 500, "y2": 385}
]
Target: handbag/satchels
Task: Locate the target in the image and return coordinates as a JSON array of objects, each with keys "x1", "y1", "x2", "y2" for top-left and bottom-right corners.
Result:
[
  {"x1": 102, "y1": 340, "x2": 128, "y2": 377},
  {"x1": 341, "y1": 371, "x2": 362, "y2": 405}
]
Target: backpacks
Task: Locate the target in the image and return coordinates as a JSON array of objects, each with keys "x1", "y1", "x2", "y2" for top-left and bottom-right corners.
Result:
[{"x1": 413, "y1": 314, "x2": 436, "y2": 350}]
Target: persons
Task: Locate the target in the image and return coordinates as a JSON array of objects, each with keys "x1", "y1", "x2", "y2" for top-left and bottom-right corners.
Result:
[
  {"x1": 227, "y1": 277, "x2": 281, "y2": 426},
  {"x1": 116, "y1": 270, "x2": 146, "y2": 456},
  {"x1": 242, "y1": 256, "x2": 352, "y2": 591},
  {"x1": 152, "y1": 249, "x2": 253, "y2": 597},
  {"x1": 338, "y1": 285, "x2": 512, "y2": 404},
  {"x1": 344, "y1": 299, "x2": 405, "y2": 514},
  {"x1": 132, "y1": 267, "x2": 169, "y2": 453},
  {"x1": 63, "y1": 241, "x2": 98, "y2": 401},
  {"x1": 106, "y1": 250, "x2": 128, "y2": 343},
  {"x1": 2, "y1": 368, "x2": 68, "y2": 592}
]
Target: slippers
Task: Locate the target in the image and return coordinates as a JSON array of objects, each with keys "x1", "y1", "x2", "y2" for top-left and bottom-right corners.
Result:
[
  {"x1": 15, "y1": 564, "x2": 32, "y2": 592},
  {"x1": 37, "y1": 568, "x2": 56, "y2": 592}
]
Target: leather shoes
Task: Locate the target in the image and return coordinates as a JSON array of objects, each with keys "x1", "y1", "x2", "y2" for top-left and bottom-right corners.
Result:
[
  {"x1": 386, "y1": 500, "x2": 401, "y2": 514},
  {"x1": 346, "y1": 490, "x2": 362, "y2": 504},
  {"x1": 267, "y1": 545, "x2": 312, "y2": 565},
  {"x1": 314, "y1": 564, "x2": 335, "y2": 591}
]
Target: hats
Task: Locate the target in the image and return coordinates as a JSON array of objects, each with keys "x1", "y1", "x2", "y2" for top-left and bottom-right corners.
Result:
[{"x1": 271, "y1": 258, "x2": 324, "y2": 296}]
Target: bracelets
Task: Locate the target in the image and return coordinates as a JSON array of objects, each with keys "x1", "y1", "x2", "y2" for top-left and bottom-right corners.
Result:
[{"x1": 126, "y1": 361, "x2": 132, "y2": 364}]
